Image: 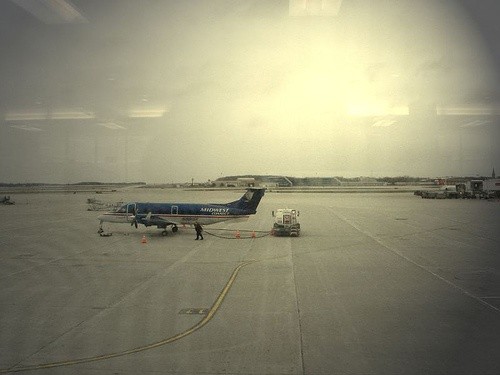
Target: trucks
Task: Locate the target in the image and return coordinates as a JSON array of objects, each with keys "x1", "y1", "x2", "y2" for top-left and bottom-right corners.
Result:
[{"x1": 272, "y1": 208, "x2": 300, "y2": 237}]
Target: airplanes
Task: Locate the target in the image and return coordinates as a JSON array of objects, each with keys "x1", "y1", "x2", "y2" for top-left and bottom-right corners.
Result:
[{"x1": 96, "y1": 188, "x2": 266, "y2": 237}]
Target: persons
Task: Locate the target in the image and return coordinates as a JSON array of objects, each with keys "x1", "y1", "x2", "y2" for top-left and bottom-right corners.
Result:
[
  {"x1": 193, "y1": 221, "x2": 204, "y2": 240},
  {"x1": 195, "y1": 222, "x2": 203, "y2": 231}
]
